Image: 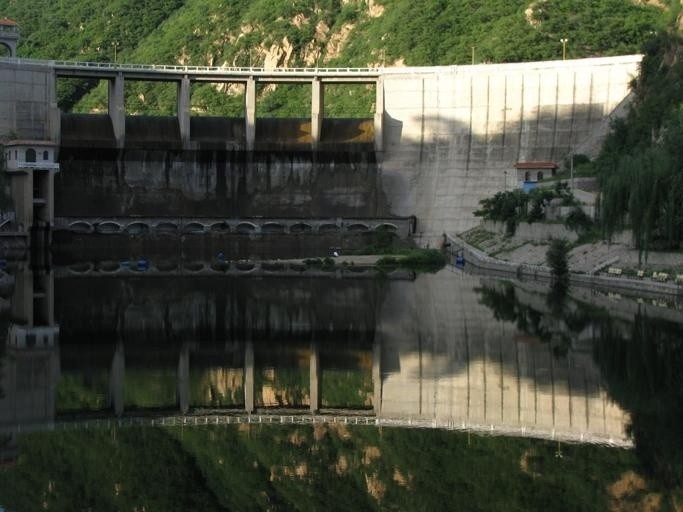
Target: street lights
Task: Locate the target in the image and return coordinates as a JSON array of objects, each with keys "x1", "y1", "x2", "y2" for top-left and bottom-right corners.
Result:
[
  {"x1": 558, "y1": 38, "x2": 568, "y2": 60},
  {"x1": 111, "y1": 39, "x2": 118, "y2": 62}
]
